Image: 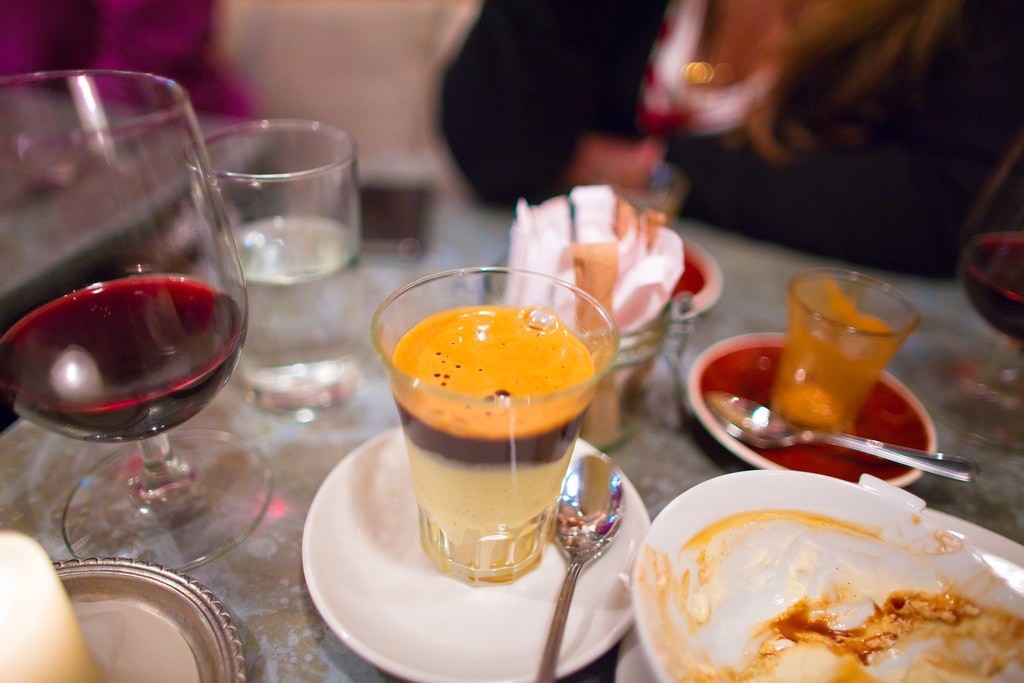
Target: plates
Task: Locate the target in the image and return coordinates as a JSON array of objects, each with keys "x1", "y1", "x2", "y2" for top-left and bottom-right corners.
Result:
[
  {"x1": 634, "y1": 469, "x2": 1024, "y2": 682},
  {"x1": 687, "y1": 333, "x2": 936, "y2": 488},
  {"x1": 299, "y1": 425, "x2": 651, "y2": 683},
  {"x1": 53, "y1": 558, "x2": 249, "y2": 683}
]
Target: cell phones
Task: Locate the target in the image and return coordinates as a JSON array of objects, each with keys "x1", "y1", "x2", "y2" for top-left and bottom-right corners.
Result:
[{"x1": 358, "y1": 179, "x2": 429, "y2": 257}]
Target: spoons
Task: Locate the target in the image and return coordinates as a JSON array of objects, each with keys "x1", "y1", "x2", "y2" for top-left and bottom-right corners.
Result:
[
  {"x1": 531, "y1": 455, "x2": 623, "y2": 681},
  {"x1": 708, "y1": 391, "x2": 982, "y2": 483}
]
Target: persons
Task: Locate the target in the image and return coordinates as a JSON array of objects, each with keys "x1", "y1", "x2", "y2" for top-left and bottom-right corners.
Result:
[{"x1": 422, "y1": 1, "x2": 1024, "y2": 289}]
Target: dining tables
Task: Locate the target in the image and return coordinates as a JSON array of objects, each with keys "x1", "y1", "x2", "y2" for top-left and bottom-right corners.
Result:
[{"x1": 0, "y1": 92, "x2": 1024, "y2": 683}]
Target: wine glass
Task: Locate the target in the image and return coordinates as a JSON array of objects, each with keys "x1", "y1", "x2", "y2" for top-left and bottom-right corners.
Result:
[
  {"x1": 923, "y1": 135, "x2": 1024, "y2": 450},
  {"x1": 3, "y1": 72, "x2": 274, "y2": 576}
]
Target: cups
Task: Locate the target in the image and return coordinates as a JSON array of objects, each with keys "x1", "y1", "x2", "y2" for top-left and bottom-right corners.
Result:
[
  {"x1": 769, "y1": 271, "x2": 922, "y2": 436},
  {"x1": 183, "y1": 121, "x2": 359, "y2": 418},
  {"x1": 370, "y1": 266, "x2": 621, "y2": 586}
]
